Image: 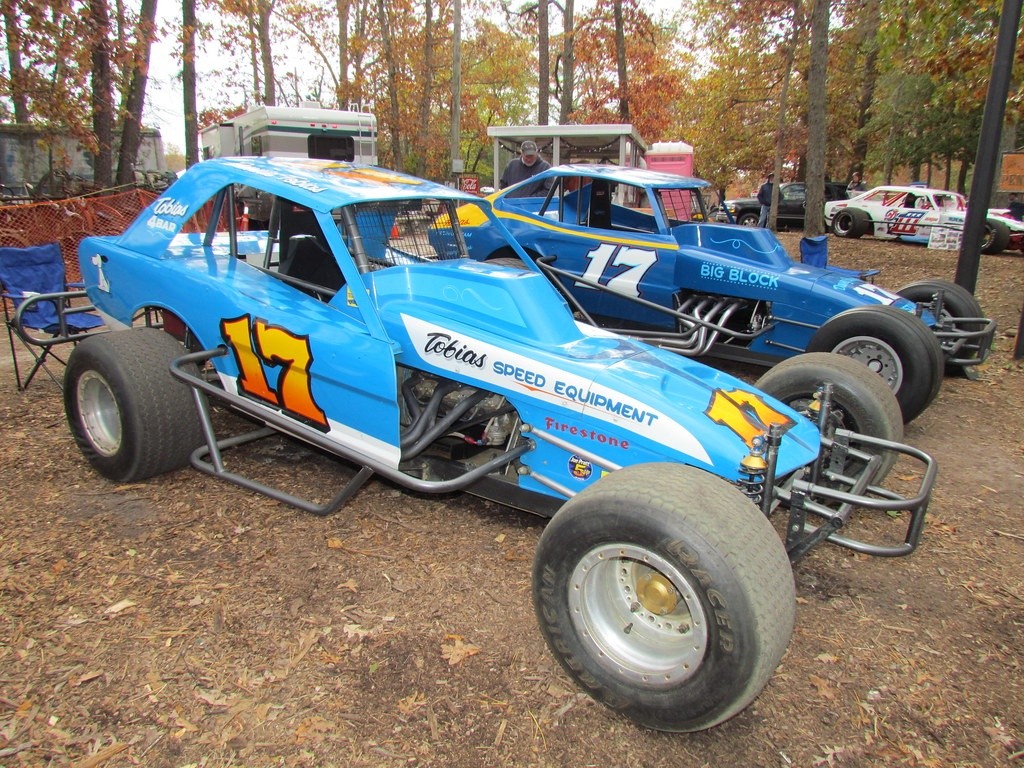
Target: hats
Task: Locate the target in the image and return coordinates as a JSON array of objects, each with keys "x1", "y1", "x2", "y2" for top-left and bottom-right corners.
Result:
[
  {"x1": 852, "y1": 172, "x2": 862, "y2": 176},
  {"x1": 768, "y1": 173, "x2": 774, "y2": 178},
  {"x1": 520, "y1": 140, "x2": 538, "y2": 157}
]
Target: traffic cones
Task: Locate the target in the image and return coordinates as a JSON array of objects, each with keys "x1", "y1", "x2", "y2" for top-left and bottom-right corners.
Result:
[
  {"x1": 240, "y1": 204, "x2": 250, "y2": 230},
  {"x1": 389, "y1": 218, "x2": 403, "y2": 240}
]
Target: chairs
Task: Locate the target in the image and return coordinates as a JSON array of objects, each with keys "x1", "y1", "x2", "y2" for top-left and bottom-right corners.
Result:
[
  {"x1": 586, "y1": 179, "x2": 612, "y2": 229},
  {"x1": 799, "y1": 236, "x2": 879, "y2": 285},
  {"x1": 0, "y1": 242, "x2": 106, "y2": 392},
  {"x1": 278, "y1": 211, "x2": 346, "y2": 303}
]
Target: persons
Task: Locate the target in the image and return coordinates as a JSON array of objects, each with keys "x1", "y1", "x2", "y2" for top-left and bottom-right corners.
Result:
[
  {"x1": 499, "y1": 141, "x2": 555, "y2": 198},
  {"x1": 757, "y1": 173, "x2": 780, "y2": 229},
  {"x1": 845, "y1": 172, "x2": 867, "y2": 200},
  {"x1": 1008, "y1": 193, "x2": 1024, "y2": 222}
]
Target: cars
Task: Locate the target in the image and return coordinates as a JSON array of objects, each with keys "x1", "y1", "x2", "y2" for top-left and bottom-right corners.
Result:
[
  {"x1": 824, "y1": 186, "x2": 1024, "y2": 254},
  {"x1": 716, "y1": 182, "x2": 851, "y2": 228},
  {"x1": 16, "y1": 155, "x2": 937, "y2": 732},
  {"x1": 425, "y1": 162, "x2": 997, "y2": 426}
]
